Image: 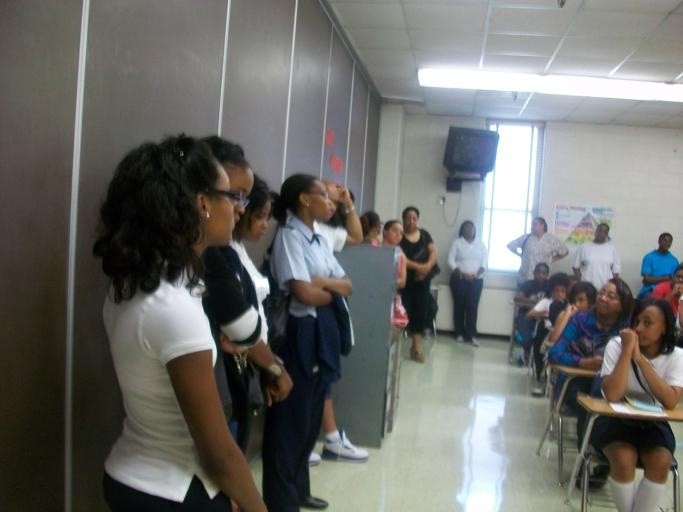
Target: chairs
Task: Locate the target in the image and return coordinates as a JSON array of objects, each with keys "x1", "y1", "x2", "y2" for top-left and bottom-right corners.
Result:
[{"x1": 402, "y1": 289, "x2": 438, "y2": 341}]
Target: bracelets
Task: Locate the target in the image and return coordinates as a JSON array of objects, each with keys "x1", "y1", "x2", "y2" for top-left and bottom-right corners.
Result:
[
  {"x1": 343, "y1": 205, "x2": 354, "y2": 216},
  {"x1": 261, "y1": 352, "x2": 285, "y2": 386}
]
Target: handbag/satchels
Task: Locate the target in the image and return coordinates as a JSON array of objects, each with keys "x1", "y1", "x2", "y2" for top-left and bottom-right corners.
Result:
[{"x1": 258, "y1": 263, "x2": 289, "y2": 341}]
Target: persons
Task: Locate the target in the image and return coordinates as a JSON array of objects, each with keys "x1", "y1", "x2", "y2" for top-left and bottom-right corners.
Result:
[
  {"x1": 92, "y1": 130, "x2": 272, "y2": 512},
  {"x1": 231, "y1": 173, "x2": 271, "y2": 395},
  {"x1": 359, "y1": 205, "x2": 443, "y2": 365},
  {"x1": 506, "y1": 217, "x2": 623, "y2": 397},
  {"x1": 194, "y1": 136, "x2": 294, "y2": 459},
  {"x1": 308, "y1": 179, "x2": 369, "y2": 467},
  {"x1": 270, "y1": 172, "x2": 354, "y2": 512},
  {"x1": 547, "y1": 276, "x2": 634, "y2": 492},
  {"x1": 447, "y1": 221, "x2": 489, "y2": 348},
  {"x1": 329, "y1": 188, "x2": 356, "y2": 230},
  {"x1": 637, "y1": 232, "x2": 683, "y2": 348},
  {"x1": 593, "y1": 297, "x2": 681, "y2": 511}
]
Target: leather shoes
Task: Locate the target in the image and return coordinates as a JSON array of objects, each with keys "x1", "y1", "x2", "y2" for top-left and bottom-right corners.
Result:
[{"x1": 303, "y1": 497, "x2": 328, "y2": 509}]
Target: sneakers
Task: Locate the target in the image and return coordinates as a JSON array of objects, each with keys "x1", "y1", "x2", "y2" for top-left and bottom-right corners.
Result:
[
  {"x1": 307, "y1": 452, "x2": 321, "y2": 466},
  {"x1": 456, "y1": 336, "x2": 464, "y2": 343},
  {"x1": 470, "y1": 338, "x2": 479, "y2": 347},
  {"x1": 321, "y1": 427, "x2": 368, "y2": 461}
]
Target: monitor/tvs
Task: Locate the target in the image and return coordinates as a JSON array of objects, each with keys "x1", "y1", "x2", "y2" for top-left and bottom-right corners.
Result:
[{"x1": 443, "y1": 126, "x2": 500, "y2": 172}]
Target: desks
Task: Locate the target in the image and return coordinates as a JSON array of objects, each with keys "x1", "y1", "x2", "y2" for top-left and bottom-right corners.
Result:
[{"x1": 507, "y1": 296, "x2": 681, "y2": 512}]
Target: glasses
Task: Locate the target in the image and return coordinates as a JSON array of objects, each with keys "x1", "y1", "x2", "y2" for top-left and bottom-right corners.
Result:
[
  {"x1": 215, "y1": 190, "x2": 244, "y2": 204},
  {"x1": 304, "y1": 189, "x2": 329, "y2": 199}
]
